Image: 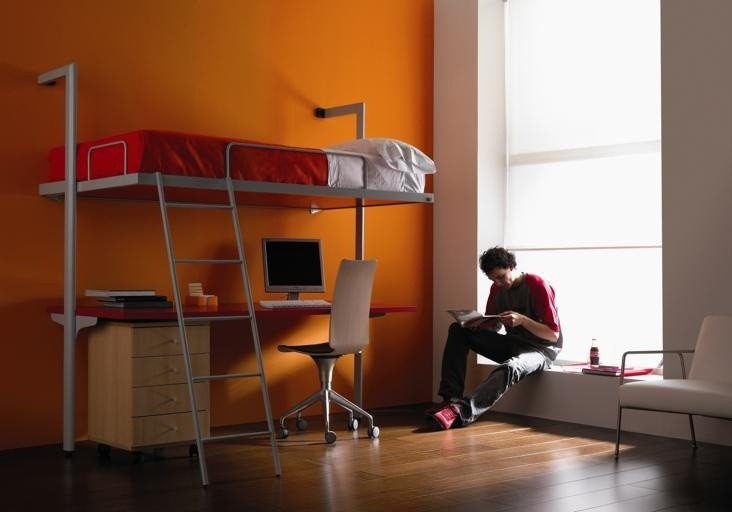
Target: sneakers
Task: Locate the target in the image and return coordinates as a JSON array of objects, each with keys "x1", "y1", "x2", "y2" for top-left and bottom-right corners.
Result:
[{"x1": 425, "y1": 400, "x2": 459, "y2": 430}]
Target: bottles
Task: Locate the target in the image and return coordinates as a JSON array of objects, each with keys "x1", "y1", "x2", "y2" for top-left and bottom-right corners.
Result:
[{"x1": 590, "y1": 338, "x2": 599, "y2": 365}]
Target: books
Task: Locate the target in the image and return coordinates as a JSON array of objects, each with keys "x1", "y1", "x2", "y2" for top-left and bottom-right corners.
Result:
[
  {"x1": 101, "y1": 300, "x2": 173, "y2": 309},
  {"x1": 83, "y1": 288, "x2": 155, "y2": 299},
  {"x1": 581, "y1": 363, "x2": 631, "y2": 377},
  {"x1": 95, "y1": 295, "x2": 168, "y2": 303},
  {"x1": 447, "y1": 309, "x2": 505, "y2": 333}
]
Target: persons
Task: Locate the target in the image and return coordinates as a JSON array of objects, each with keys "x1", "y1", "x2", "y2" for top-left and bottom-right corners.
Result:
[{"x1": 425, "y1": 247, "x2": 564, "y2": 431}]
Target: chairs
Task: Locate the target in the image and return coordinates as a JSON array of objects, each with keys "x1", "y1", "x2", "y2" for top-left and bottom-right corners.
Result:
[
  {"x1": 612, "y1": 314, "x2": 730, "y2": 459},
  {"x1": 276, "y1": 259, "x2": 379, "y2": 441}
]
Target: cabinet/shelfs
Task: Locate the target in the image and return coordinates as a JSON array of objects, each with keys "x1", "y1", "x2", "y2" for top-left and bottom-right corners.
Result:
[{"x1": 81, "y1": 319, "x2": 212, "y2": 459}]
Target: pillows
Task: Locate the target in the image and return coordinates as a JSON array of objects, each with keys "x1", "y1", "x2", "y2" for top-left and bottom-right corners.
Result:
[{"x1": 327, "y1": 135, "x2": 438, "y2": 174}]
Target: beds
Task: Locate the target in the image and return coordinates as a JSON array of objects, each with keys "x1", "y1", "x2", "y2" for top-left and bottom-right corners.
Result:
[{"x1": 48, "y1": 129, "x2": 438, "y2": 210}]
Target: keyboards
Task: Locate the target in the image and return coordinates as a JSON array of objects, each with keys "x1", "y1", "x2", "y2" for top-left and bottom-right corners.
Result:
[{"x1": 259, "y1": 300, "x2": 331, "y2": 308}]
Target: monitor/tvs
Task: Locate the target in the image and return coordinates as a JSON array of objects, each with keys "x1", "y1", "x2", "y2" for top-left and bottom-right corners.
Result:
[{"x1": 262, "y1": 237, "x2": 327, "y2": 300}]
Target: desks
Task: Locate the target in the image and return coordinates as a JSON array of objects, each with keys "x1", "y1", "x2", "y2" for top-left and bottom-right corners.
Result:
[{"x1": 49, "y1": 299, "x2": 418, "y2": 465}]
